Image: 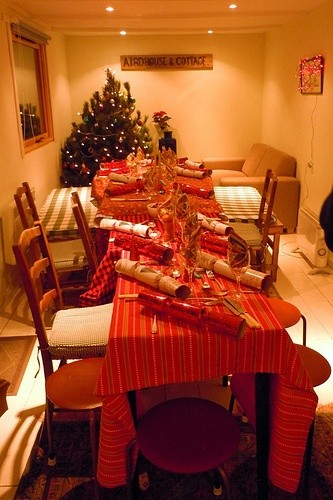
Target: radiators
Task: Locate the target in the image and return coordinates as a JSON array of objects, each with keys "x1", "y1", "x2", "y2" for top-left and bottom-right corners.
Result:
[{"x1": 290, "y1": 207, "x2": 333, "y2": 276}]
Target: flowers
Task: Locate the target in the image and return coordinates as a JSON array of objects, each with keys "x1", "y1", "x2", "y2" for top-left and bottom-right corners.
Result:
[{"x1": 152, "y1": 111, "x2": 172, "y2": 131}]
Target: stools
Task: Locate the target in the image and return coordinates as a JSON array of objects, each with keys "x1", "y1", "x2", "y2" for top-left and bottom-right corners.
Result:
[
  {"x1": 228, "y1": 344, "x2": 331, "y2": 500},
  {"x1": 126, "y1": 397, "x2": 240, "y2": 500},
  {"x1": 46, "y1": 358, "x2": 105, "y2": 478},
  {"x1": 268, "y1": 298, "x2": 306, "y2": 346}
]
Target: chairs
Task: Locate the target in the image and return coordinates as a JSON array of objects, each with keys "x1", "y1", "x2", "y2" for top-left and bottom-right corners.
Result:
[{"x1": 12, "y1": 168, "x2": 279, "y2": 447}]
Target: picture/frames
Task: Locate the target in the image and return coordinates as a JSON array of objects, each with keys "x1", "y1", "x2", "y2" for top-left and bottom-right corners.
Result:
[{"x1": 300, "y1": 56, "x2": 323, "y2": 94}]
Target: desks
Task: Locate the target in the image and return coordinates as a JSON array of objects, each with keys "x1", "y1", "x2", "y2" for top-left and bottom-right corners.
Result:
[
  {"x1": 39, "y1": 187, "x2": 98, "y2": 294},
  {"x1": 97, "y1": 249, "x2": 319, "y2": 500},
  {"x1": 91, "y1": 159, "x2": 228, "y2": 256},
  {"x1": 80, "y1": 216, "x2": 175, "y2": 302},
  {"x1": 214, "y1": 185, "x2": 284, "y2": 283}
]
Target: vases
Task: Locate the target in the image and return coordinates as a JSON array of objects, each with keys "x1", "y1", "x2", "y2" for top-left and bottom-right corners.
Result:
[{"x1": 159, "y1": 132, "x2": 176, "y2": 154}]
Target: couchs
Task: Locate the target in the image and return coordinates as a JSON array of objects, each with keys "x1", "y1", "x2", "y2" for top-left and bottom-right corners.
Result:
[{"x1": 195, "y1": 143, "x2": 300, "y2": 234}]
